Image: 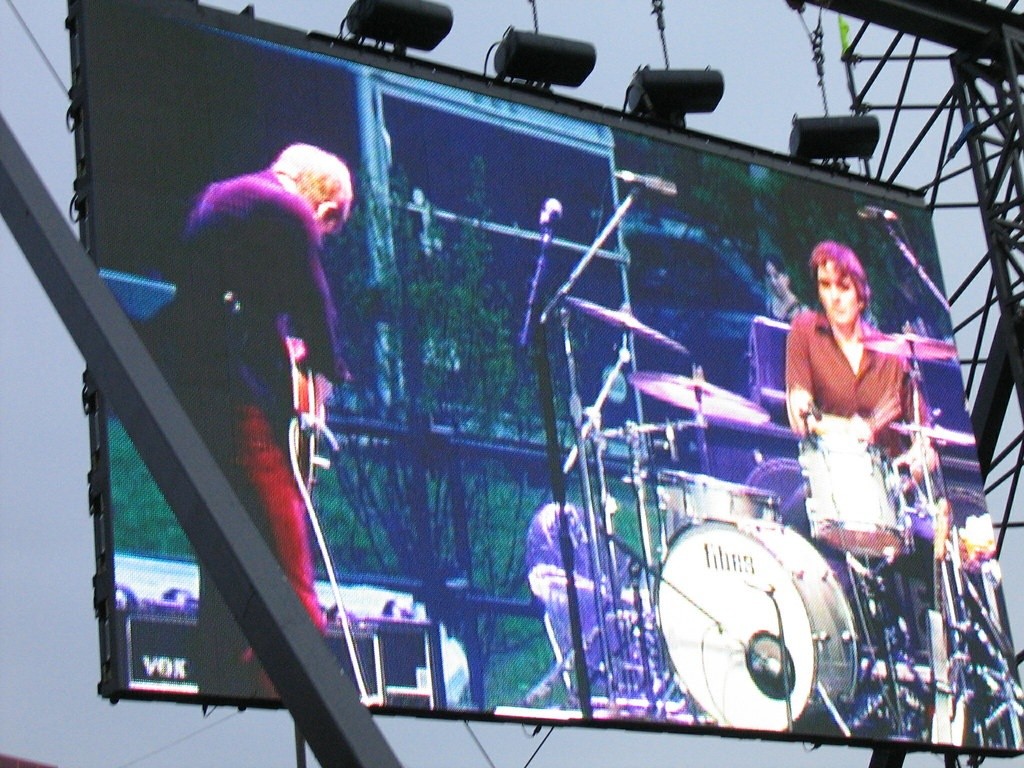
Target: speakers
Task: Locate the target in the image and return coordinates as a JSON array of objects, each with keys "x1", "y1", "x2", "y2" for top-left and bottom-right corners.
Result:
[
  {"x1": 790, "y1": 115, "x2": 880, "y2": 165},
  {"x1": 495, "y1": 30, "x2": 596, "y2": 89},
  {"x1": 344, "y1": 0, "x2": 452, "y2": 51},
  {"x1": 625, "y1": 68, "x2": 725, "y2": 115},
  {"x1": 120, "y1": 598, "x2": 447, "y2": 714}
]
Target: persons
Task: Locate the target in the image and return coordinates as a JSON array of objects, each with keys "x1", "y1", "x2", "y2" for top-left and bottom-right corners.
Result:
[
  {"x1": 182, "y1": 142, "x2": 353, "y2": 634},
  {"x1": 761, "y1": 255, "x2": 807, "y2": 320},
  {"x1": 786, "y1": 242, "x2": 939, "y2": 661}
]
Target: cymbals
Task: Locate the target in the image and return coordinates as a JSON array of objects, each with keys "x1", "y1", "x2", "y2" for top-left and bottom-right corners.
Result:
[
  {"x1": 568, "y1": 296, "x2": 693, "y2": 356},
  {"x1": 858, "y1": 334, "x2": 956, "y2": 363},
  {"x1": 626, "y1": 372, "x2": 772, "y2": 425},
  {"x1": 893, "y1": 422, "x2": 975, "y2": 446}
]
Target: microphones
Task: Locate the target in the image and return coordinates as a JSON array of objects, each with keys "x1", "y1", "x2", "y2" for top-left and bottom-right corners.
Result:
[
  {"x1": 617, "y1": 172, "x2": 678, "y2": 197},
  {"x1": 858, "y1": 206, "x2": 900, "y2": 223},
  {"x1": 537, "y1": 197, "x2": 563, "y2": 267}
]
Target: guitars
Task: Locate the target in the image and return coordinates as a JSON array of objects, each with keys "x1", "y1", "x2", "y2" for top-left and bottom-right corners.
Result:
[{"x1": 285, "y1": 333, "x2": 319, "y2": 488}]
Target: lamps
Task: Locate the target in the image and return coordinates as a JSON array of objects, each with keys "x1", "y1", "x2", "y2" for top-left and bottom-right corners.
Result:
[
  {"x1": 790, "y1": 113, "x2": 881, "y2": 165},
  {"x1": 627, "y1": 63, "x2": 724, "y2": 129},
  {"x1": 337, "y1": 0, "x2": 454, "y2": 58},
  {"x1": 489, "y1": 27, "x2": 596, "y2": 93}
]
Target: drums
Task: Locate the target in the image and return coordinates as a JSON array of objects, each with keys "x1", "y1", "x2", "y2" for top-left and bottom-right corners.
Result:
[
  {"x1": 655, "y1": 470, "x2": 783, "y2": 567},
  {"x1": 657, "y1": 515, "x2": 859, "y2": 734}
]
